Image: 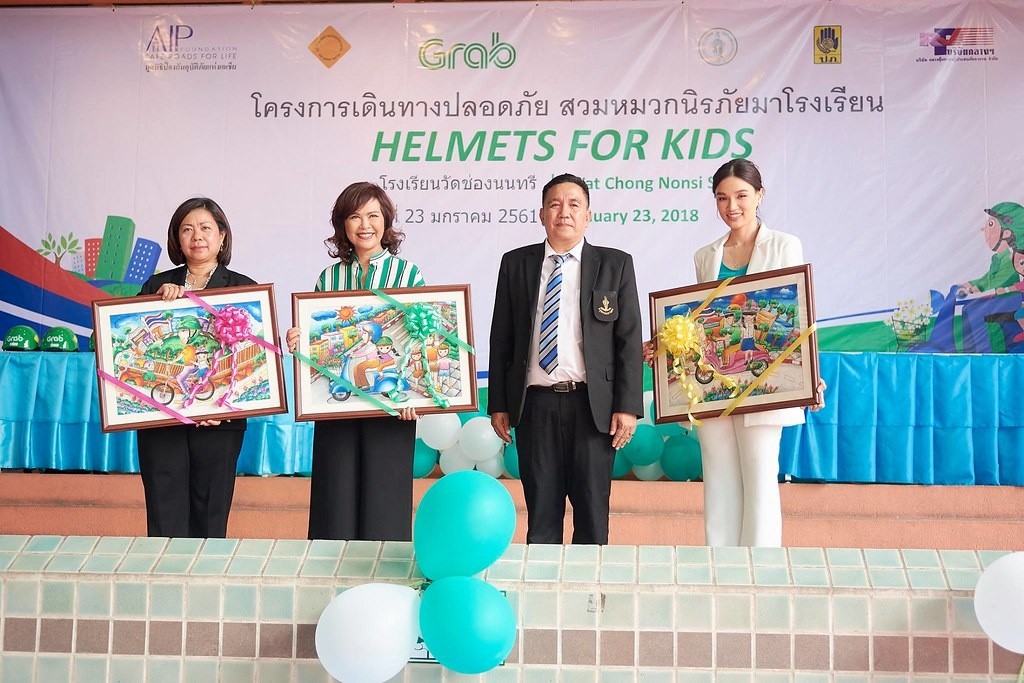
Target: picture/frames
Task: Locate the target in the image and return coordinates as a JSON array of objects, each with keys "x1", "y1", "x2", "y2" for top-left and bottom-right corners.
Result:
[
  {"x1": 92, "y1": 282, "x2": 288, "y2": 435},
  {"x1": 290, "y1": 284, "x2": 479, "y2": 422},
  {"x1": 649, "y1": 263, "x2": 821, "y2": 425}
]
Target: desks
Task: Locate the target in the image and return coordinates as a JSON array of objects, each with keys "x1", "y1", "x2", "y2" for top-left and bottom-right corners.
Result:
[
  {"x1": 778, "y1": 349, "x2": 1024, "y2": 486},
  {"x1": 0, "y1": 349, "x2": 315, "y2": 477}
]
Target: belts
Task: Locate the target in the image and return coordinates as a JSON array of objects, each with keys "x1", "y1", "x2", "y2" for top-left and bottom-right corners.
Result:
[{"x1": 548, "y1": 382, "x2": 585, "y2": 393}]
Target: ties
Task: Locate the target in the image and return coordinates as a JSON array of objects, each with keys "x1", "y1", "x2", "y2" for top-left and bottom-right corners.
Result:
[{"x1": 539, "y1": 254, "x2": 571, "y2": 375}]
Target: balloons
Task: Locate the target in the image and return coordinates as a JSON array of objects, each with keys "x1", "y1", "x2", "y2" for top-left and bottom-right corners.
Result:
[
  {"x1": 413, "y1": 404, "x2": 523, "y2": 479},
  {"x1": 610, "y1": 392, "x2": 705, "y2": 480},
  {"x1": 314, "y1": 583, "x2": 422, "y2": 683},
  {"x1": 973, "y1": 550, "x2": 1024, "y2": 653},
  {"x1": 413, "y1": 468, "x2": 517, "y2": 583},
  {"x1": 419, "y1": 577, "x2": 518, "y2": 675}
]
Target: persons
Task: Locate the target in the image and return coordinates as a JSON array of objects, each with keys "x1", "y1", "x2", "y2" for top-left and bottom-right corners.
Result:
[
  {"x1": 135, "y1": 197, "x2": 258, "y2": 539},
  {"x1": 640, "y1": 158, "x2": 828, "y2": 548},
  {"x1": 285, "y1": 181, "x2": 426, "y2": 543},
  {"x1": 486, "y1": 174, "x2": 646, "y2": 545}
]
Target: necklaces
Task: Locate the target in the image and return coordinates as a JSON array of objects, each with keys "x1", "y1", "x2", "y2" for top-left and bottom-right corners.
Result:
[
  {"x1": 186, "y1": 266, "x2": 216, "y2": 291},
  {"x1": 732, "y1": 253, "x2": 745, "y2": 268}
]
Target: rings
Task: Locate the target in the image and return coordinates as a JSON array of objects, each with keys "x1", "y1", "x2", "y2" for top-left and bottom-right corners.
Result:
[
  {"x1": 168, "y1": 285, "x2": 173, "y2": 289},
  {"x1": 628, "y1": 433, "x2": 632, "y2": 436}
]
